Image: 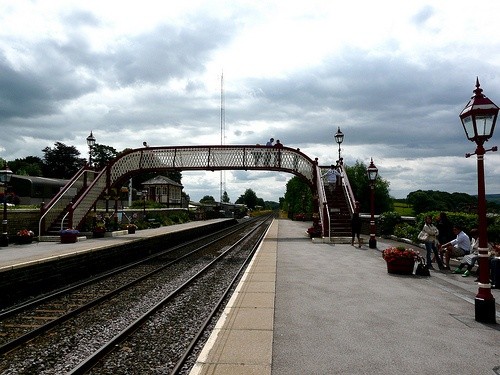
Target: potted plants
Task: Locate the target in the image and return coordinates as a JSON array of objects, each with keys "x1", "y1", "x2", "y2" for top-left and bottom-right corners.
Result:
[
  {"x1": 120, "y1": 224, "x2": 129, "y2": 235},
  {"x1": 104, "y1": 214, "x2": 115, "y2": 237}
]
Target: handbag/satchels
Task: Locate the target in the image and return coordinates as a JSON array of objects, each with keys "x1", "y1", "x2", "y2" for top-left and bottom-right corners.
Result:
[
  {"x1": 417, "y1": 230, "x2": 428, "y2": 241},
  {"x1": 414, "y1": 259, "x2": 430, "y2": 276}
]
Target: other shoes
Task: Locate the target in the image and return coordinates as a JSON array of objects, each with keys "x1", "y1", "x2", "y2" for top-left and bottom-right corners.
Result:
[
  {"x1": 462, "y1": 269, "x2": 469, "y2": 277},
  {"x1": 451, "y1": 267, "x2": 462, "y2": 274}
]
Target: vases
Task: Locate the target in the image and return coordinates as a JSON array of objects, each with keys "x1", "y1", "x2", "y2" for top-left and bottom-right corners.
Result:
[
  {"x1": 60, "y1": 233, "x2": 80, "y2": 244},
  {"x1": 128, "y1": 228, "x2": 135, "y2": 234},
  {"x1": 386, "y1": 258, "x2": 415, "y2": 275},
  {"x1": 92, "y1": 231, "x2": 105, "y2": 239},
  {"x1": 17, "y1": 235, "x2": 33, "y2": 245}
]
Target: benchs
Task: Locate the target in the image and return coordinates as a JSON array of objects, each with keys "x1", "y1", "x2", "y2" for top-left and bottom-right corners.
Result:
[{"x1": 438, "y1": 253, "x2": 479, "y2": 268}]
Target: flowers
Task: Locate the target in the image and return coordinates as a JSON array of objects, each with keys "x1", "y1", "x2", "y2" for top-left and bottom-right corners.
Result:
[
  {"x1": 93, "y1": 215, "x2": 107, "y2": 234},
  {"x1": 126, "y1": 223, "x2": 139, "y2": 230},
  {"x1": 382, "y1": 246, "x2": 420, "y2": 263},
  {"x1": 16, "y1": 229, "x2": 35, "y2": 236},
  {"x1": 0, "y1": 193, "x2": 16, "y2": 205},
  {"x1": 58, "y1": 228, "x2": 81, "y2": 235}
]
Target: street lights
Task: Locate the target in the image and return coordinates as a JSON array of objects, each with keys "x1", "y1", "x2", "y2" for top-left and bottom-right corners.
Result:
[
  {"x1": 459, "y1": 76, "x2": 500, "y2": 326},
  {"x1": 367, "y1": 158, "x2": 377, "y2": 249},
  {"x1": 334, "y1": 125, "x2": 345, "y2": 159},
  {"x1": 86, "y1": 131, "x2": 96, "y2": 167}
]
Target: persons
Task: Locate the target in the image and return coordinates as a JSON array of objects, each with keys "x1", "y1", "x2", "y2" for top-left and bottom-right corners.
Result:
[
  {"x1": 321, "y1": 165, "x2": 344, "y2": 195},
  {"x1": 263, "y1": 138, "x2": 274, "y2": 166},
  {"x1": 350, "y1": 208, "x2": 361, "y2": 247},
  {"x1": 272, "y1": 140, "x2": 283, "y2": 167},
  {"x1": 473, "y1": 243, "x2": 500, "y2": 289},
  {"x1": 440, "y1": 223, "x2": 471, "y2": 270},
  {"x1": 251, "y1": 144, "x2": 263, "y2": 166},
  {"x1": 423, "y1": 215, "x2": 445, "y2": 270},
  {"x1": 292, "y1": 148, "x2": 300, "y2": 172},
  {"x1": 452, "y1": 229, "x2": 479, "y2": 277},
  {"x1": 143, "y1": 142, "x2": 153, "y2": 166}
]
